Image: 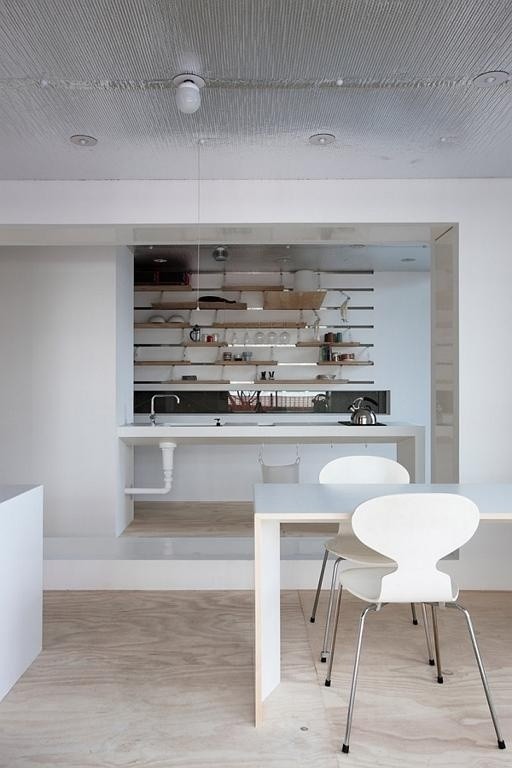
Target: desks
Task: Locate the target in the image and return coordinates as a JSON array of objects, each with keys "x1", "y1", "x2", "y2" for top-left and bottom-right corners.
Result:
[{"x1": 251, "y1": 483, "x2": 511, "y2": 731}]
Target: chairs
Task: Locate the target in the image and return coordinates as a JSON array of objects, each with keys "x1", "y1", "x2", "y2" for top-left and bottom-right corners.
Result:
[
  {"x1": 325, "y1": 493, "x2": 506, "y2": 753},
  {"x1": 310, "y1": 455, "x2": 436, "y2": 666}
]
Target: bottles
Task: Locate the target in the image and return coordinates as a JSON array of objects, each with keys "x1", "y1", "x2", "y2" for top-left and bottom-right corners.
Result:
[
  {"x1": 326, "y1": 345, "x2": 332, "y2": 361},
  {"x1": 190, "y1": 324, "x2": 218, "y2": 342},
  {"x1": 224, "y1": 352, "x2": 232, "y2": 361}
]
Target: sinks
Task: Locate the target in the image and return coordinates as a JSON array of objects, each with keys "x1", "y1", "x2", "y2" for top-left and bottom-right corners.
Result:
[{"x1": 167, "y1": 423, "x2": 225, "y2": 426}]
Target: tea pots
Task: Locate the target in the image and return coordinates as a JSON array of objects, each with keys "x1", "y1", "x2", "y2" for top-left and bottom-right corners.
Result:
[
  {"x1": 312, "y1": 394, "x2": 330, "y2": 412},
  {"x1": 348, "y1": 395, "x2": 379, "y2": 426}
]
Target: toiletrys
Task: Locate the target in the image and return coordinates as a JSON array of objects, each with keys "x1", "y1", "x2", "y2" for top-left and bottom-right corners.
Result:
[{"x1": 214, "y1": 419, "x2": 220, "y2": 426}]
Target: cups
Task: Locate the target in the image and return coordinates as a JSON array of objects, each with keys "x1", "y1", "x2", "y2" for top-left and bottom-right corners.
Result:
[{"x1": 234, "y1": 352, "x2": 252, "y2": 361}]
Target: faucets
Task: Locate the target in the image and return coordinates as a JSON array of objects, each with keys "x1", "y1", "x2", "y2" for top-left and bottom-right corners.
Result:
[{"x1": 151, "y1": 394, "x2": 180, "y2": 414}]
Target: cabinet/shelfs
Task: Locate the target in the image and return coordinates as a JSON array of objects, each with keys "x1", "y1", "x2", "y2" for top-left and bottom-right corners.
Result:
[{"x1": 135, "y1": 268, "x2": 375, "y2": 386}]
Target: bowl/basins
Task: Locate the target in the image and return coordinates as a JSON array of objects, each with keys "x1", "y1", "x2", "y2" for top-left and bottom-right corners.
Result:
[
  {"x1": 168, "y1": 314, "x2": 185, "y2": 323},
  {"x1": 148, "y1": 315, "x2": 166, "y2": 323}
]
